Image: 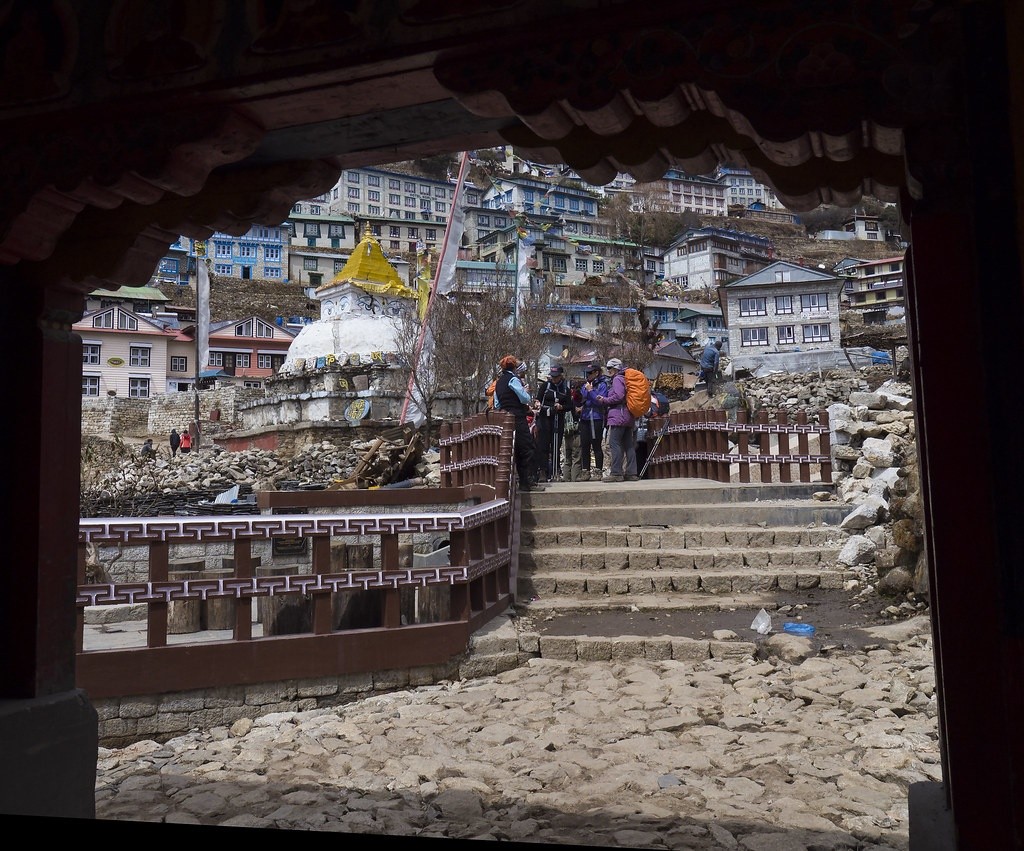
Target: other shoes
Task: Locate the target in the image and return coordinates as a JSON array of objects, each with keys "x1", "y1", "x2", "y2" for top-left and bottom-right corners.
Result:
[
  {"x1": 708, "y1": 394, "x2": 714, "y2": 398},
  {"x1": 518, "y1": 479, "x2": 545, "y2": 491}
]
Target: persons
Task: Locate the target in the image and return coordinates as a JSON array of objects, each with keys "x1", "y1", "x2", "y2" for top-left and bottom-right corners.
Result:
[
  {"x1": 141, "y1": 439, "x2": 157, "y2": 462},
  {"x1": 170, "y1": 428, "x2": 192, "y2": 459},
  {"x1": 700, "y1": 341, "x2": 723, "y2": 398},
  {"x1": 486, "y1": 354, "x2": 669, "y2": 492}
]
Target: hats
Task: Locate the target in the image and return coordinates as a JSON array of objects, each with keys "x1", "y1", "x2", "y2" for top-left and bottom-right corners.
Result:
[
  {"x1": 583, "y1": 364, "x2": 599, "y2": 372},
  {"x1": 607, "y1": 358, "x2": 623, "y2": 370},
  {"x1": 548, "y1": 365, "x2": 563, "y2": 377}
]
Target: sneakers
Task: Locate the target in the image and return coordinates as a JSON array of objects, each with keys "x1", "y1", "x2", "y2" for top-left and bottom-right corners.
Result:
[
  {"x1": 576, "y1": 470, "x2": 590, "y2": 481},
  {"x1": 601, "y1": 474, "x2": 622, "y2": 482},
  {"x1": 589, "y1": 467, "x2": 603, "y2": 481},
  {"x1": 624, "y1": 475, "x2": 639, "y2": 481},
  {"x1": 539, "y1": 471, "x2": 546, "y2": 482}
]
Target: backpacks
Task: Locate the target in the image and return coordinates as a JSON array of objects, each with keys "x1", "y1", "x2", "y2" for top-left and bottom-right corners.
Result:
[{"x1": 624, "y1": 368, "x2": 651, "y2": 417}]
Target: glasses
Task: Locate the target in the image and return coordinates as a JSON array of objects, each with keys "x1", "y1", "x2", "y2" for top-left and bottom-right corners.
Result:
[
  {"x1": 587, "y1": 372, "x2": 593, "y2": 374},
  {"x1": 607, "y1": 366, "x2": 613, "y2": 369}
]
